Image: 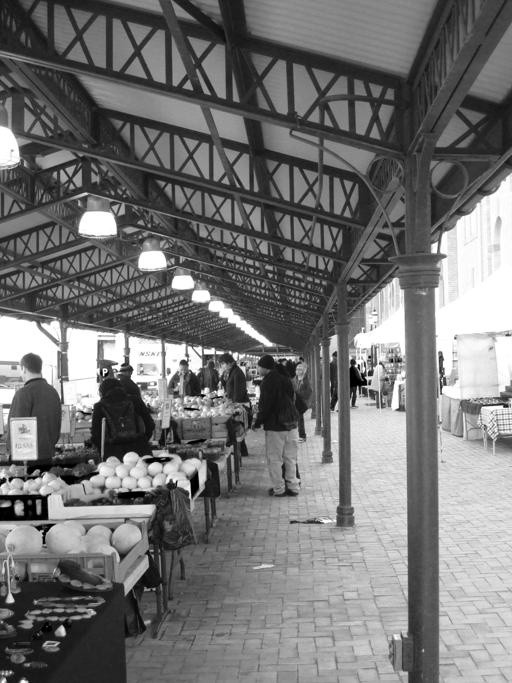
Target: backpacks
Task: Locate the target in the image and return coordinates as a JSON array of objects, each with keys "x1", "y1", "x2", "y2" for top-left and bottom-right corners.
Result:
[{"x1": 102, "y1": 400, "x2": 144, "y2": 443}]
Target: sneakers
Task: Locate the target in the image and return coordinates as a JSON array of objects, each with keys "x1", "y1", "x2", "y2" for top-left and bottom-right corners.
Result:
[{"x1": 268, "y1": 486, "x2": 301, "y2": 498}]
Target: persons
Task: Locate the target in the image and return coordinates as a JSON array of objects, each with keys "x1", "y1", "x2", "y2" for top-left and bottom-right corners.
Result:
[
  {"x1": 370, "y1": 361, "x2": 389, "y2": 408},
  {"x1": 116, "y1": 361, "x2": 141, "y2": 396},
  {"x1": 83, "y1": 377, "x2": 153, "y2": 463},
  {"x1": 167, "y1": 351, "x2": 365, "y2": 497},
  {"x1": 438, "y1": 350, "x2": 445, "y2": 394},
  {"x1": 4, "y1": 351, "x2": 64, "y2": 460}
]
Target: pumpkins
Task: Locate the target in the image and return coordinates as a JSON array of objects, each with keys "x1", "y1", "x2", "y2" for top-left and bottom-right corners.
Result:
[
  {"x1": 4, "y1": 521, "x2": 141, "y2": 563},
  {"x1": 0, "y1": 451, "x2": 201, "y2": 496}
]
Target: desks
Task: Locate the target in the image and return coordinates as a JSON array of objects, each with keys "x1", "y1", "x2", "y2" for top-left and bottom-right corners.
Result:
[{"x1": 344, "y1": 342, "x2": 512, "y2": 456}]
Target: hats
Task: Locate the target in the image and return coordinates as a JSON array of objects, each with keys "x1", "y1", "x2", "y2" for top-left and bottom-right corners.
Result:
[{"x1": 258, "y1": 355, "x2": 275, "y2": 369}]
